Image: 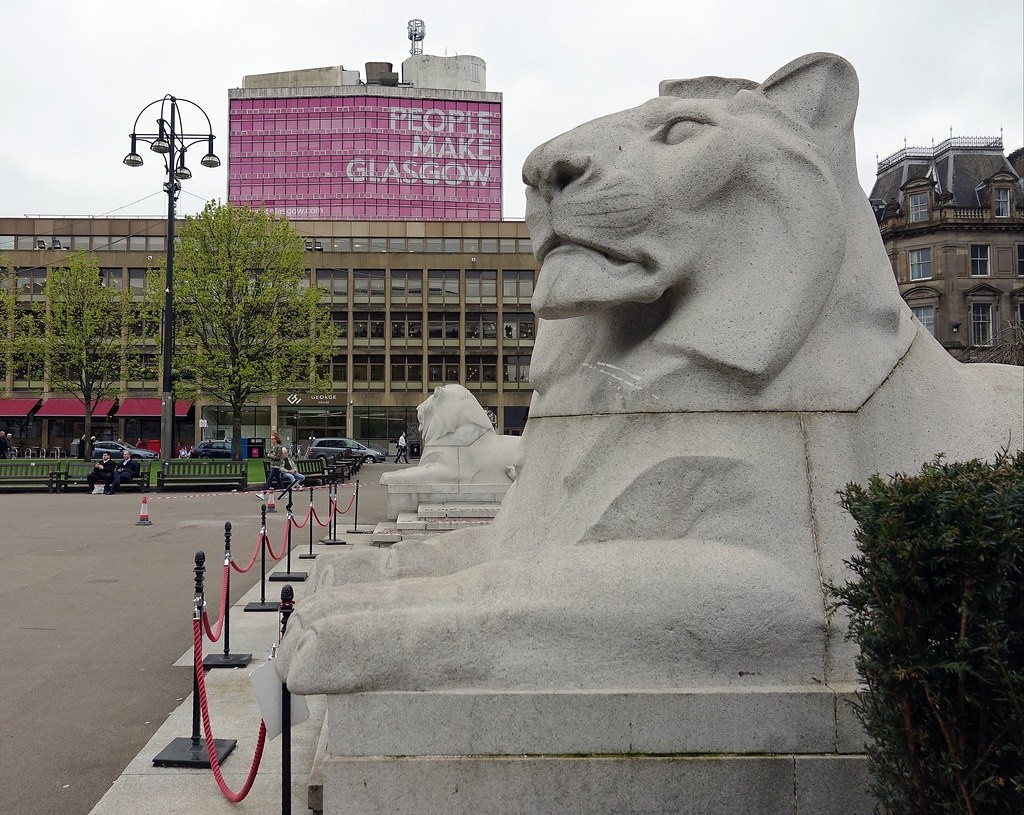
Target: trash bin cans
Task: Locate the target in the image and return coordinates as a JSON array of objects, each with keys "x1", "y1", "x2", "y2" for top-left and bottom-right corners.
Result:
[
  {"x1": 408, "y1": 440, "x2": 421, "y2": 459},
  {"x1": 77, "y1": 439, "x2": 94, "y2": 459},
  {"x1": 147, "y1": 439, "x2": 160, "y2": 455},
  {"x1": 247, "y1": 438, "x2": 266, "y2": 459},
  {"x1": 231, "y1": 438, "x2": 248, "y2": 459}
]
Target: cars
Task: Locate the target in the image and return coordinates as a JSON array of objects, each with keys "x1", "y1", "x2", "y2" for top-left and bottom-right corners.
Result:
[{"x1": 90, "y1": 440, "x2": 160, "y2": 460}]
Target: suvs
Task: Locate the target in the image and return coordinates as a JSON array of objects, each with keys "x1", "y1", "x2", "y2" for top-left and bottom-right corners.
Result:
[
  {"x1": 187, "y1": 437, "x2": 232, "y2": 459},
  {"x1": 306, "y1": 437, "x2": 386, "y2": 463}
]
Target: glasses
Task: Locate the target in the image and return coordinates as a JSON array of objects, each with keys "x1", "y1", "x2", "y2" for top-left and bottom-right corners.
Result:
[{"x1": 271, "y1": 438, "x2": 276, "y2": 440}]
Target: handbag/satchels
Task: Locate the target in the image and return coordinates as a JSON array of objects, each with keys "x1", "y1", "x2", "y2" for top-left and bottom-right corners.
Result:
[{"x1": 92, "y1": 480, "x2": 105, "y2": 494}]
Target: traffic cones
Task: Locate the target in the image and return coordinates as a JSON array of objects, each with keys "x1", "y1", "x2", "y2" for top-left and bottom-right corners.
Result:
[{"x1": 135, "y1": 495, "x2": 153, "y2": 526}]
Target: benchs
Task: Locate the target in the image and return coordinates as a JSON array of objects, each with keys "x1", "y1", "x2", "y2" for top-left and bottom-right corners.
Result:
[
  {"x1": 333, "y1": 453, "x2": 357, "y2": 476},
  {"x1": 263, "y1": 457, "x2": 338, "y2": 490},
  {"x1": 346, "y1": 447, "x2": 364, "y2": 466},
  {"x1": 340, "y1": 450, "x2": 361, "y2": 476},
  {"x1": 155, "y1": 456, "x2": 252, "y2": 495},
  {"x1": 324, "y1": 455, "x2": 353, "y2": 484},
  {"x1": 56, "y1": 458, "x2": 154, "y2": 493},
  {"x1": 0, "y1": 461, "x2": 61, "y2": 495}
]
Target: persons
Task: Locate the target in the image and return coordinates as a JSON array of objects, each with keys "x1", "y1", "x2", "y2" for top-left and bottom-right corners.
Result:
[
  {"x1": 86, "y1": 451, "x2": 116, "y2": 494},
  {"x1": 183, "y1": 446, "x2": 188, "y2": 458},
  {"x1": 394, "y1": 431, "x2": 411, "y2": 464},
  {"x1": 118, "y1": 438, "x2": 123, "y2": 442},
  {"x1": 0, "y1": 431, "x2": 13, "y2": 459},
  {"x1": 5, "y1": 433, "x2": 14, "y2": 459},
  {"x1": 106, "y1": 451, "x2": 140, "y2": 495},
  {"x1": 175, "y1": 441, "x2": 183, "y2": 457},
  {"x1": 135, "y1": 437, "x2": 143, "y2": 449},
  {"x1": 278, "y1": 446, "x2": 305, "y2": 488},
  {"x1": 77, "y1": 434, "x2": 86, "y2": 460},
  {"x1": 91, "y1": 435, "x2": 96, "y2": 459},
  {"x1": 255, "y1": 432, "x2": 288, "y2": 500},
  {"x1": 190, "y1": 445, "x2": 196, "y2": 457}
]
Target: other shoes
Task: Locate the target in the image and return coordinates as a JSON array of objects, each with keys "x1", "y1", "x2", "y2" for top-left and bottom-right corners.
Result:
[
  {"x1": 106, "y1": 491, "x2": 115, "y2": 495},
  {"x1": 86, "y1": 491, "x2": 92, "y2": 494},
  {"x1": 115, "y1": 481, "x2": 120, "y2": 489},
  {"x1": 255, "y1": 493, "x2": 265, "y2": 500},
  {"x1": 406, "y1": 462, "x2": 411, "y2": 464},
  {"x1": 291, "y1": 487, "x2": 296, "y2": 491},
  {"x1": 299, "y1": 486, "x2": 305, "y2": 492},
  {"x1": 283, "y1": 489, "x2": 287, "y2": 498},
  {"x1": 394, "y1": 461, "x2": 397, "y2": 463},
  {"x1": 400, "y1": 461, "x2": 404, "y2": 464}
]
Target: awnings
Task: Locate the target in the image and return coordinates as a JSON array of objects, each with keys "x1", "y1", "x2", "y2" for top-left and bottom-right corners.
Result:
[
  {"x1": 34, "y1": 397, "x2": 116, "y2": 418},
  {"x1": 113, "y1": 397, "x2": 196, "y2": 418},
  {"x1": 0, "y1": 397, "x2": 41, "y2": 417}
]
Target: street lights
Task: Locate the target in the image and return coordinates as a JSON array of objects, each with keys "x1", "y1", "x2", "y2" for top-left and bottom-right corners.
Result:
[{"x1": 123, "y1": 93, "x2": 221, "y2": 460}]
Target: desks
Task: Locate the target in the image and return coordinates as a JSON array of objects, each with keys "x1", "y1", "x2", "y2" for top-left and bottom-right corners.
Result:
[
  {"x1": 32, "y1": 446, "x2": 41, "y2": 459},
  {"x1": 53, "y1": 446, "x2": 63, "y2": 458},
  {"x1": 12, "y1": 447, "x2": 19, "y2": 458}
]
